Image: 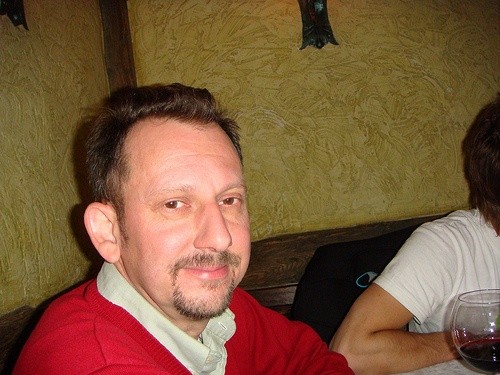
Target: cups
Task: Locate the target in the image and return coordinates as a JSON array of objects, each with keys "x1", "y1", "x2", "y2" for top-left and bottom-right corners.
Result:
[{"x1": 450, "y1": 287, "x2": 499, "y2": 375}]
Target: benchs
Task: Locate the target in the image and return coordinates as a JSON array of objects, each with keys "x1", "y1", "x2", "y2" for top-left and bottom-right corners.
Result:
[{"x1": 235, "y1": 213, "x2": 447, "y2": 316}]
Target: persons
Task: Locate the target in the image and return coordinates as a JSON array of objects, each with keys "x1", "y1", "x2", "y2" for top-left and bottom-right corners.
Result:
[
  {"x1": 329, "y1": 91, "x2": 500, "y2": 375},
  {"x1": 11, "y1": 83, "x2": 356, "y2": 375}
]
